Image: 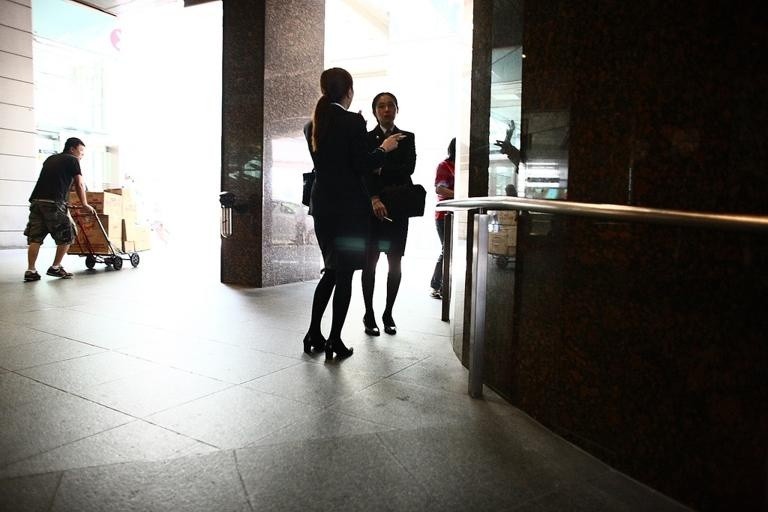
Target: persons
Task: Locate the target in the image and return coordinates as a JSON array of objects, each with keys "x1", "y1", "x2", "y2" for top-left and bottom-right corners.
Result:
[
  {"x1": 303, "y1": 67, "x2": 403, "y2": 361},
  {"x1": 361, "y1": 92, "x2": 416, "y2": 336},
  {"x1": 432, "y1": 137, "x2": 455, "y2": 299},
  {"x1": 23, "y1": 137, "x2": 97, "y2": 281}
]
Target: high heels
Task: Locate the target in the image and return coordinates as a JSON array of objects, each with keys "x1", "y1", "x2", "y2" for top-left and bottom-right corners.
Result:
[
  {"x1": 324, "y1": 339, "x2": 353, "y2": 360},
  {"x1": 303, "y1": 332, "x2": 327, "y2": 352}
]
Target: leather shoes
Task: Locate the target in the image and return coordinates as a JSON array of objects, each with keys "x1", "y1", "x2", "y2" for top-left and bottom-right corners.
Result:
[
  {"x1": 363, "y1": 313, "x2": 380, "y2": 336},
  {"x1": 382, "y1": 314, "x2": 397, "y2": 334}
]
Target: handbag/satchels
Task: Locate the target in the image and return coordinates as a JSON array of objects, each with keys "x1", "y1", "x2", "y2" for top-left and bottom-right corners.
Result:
[
  {"x1": 303, "y1": 173, "x2": 317, "y2": 206},
  {"x1": 411, "y1": 185, "x2": 426, "y2": 216}
]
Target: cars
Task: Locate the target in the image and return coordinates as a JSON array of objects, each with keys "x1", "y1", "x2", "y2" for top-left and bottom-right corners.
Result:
[{"x1": 271, "y1": 200, "x2": 318, "y2": 245}]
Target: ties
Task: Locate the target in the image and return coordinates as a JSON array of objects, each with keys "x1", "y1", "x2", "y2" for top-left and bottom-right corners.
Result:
[{"x1": 385, "y1": 128, "x2": 391, "y2": 137}]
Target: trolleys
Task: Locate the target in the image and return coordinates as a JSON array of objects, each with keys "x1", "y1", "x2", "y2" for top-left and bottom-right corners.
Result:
[
  {"x1": 486, "y1": 253, "x2": 517, "y2": 270},
  {"x1": 60, "y1": 202, "x2": 140, "y2": 271}
]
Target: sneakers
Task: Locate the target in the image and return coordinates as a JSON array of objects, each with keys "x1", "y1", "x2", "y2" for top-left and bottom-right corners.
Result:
[
  {"x1": 430, "y1": 288, "x2": 442, "y2": 299},
  {"x1": 46, "y1": 266, "x2": 73, "y2": 278},
  {"x1": 25, "y1": 271, "x2": 41, "y2": 280}
]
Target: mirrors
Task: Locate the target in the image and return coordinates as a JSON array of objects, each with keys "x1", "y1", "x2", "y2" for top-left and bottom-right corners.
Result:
[
  {"x1": 487, "y1": 45, "x2": 522, "y2": 196},
  {"x1": 522, "y1": 45, "x2": 571, "y2": 200}
]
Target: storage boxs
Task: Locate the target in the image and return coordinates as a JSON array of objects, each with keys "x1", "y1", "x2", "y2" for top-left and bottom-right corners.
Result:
[
  {"x1": 66, "y1": 186, "x2": 151, "y2": 254},
  {"x1": 489, "y1": 210, "x2": 517, "y2": 257}
]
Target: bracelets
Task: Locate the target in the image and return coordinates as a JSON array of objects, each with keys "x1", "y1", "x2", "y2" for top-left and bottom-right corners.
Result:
[{"x1": 378, "y1": 147, "x2": 385, "y2": 152}]
